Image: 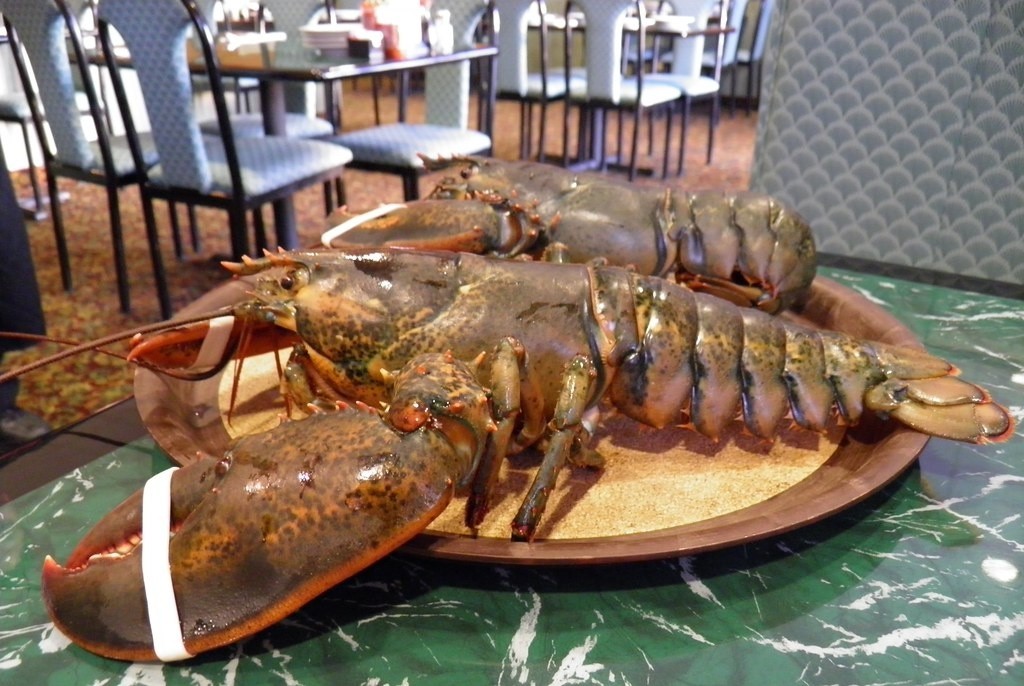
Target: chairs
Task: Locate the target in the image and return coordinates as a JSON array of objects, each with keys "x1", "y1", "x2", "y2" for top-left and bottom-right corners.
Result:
[{"x1": 0, "y1": 0, "x2": 773, "y2": 322}]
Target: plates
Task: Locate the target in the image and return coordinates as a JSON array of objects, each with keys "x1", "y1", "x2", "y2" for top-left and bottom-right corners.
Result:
[
  {"x1": 298, "y1": 23, "x2": 365, "y2": 51},
  {"x1": 223, "y1": 274, "x2": 933, "y2": 564}
]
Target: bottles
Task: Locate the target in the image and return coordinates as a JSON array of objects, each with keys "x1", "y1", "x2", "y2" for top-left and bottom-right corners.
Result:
[{"x1": 432, "y1": 9, "x2": 454, "y2": 55}]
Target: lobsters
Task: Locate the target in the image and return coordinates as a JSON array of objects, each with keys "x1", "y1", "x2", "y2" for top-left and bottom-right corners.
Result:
[
  {"x1": 0, "y1": 245, "x2": 1014, "y2": 665},
  {"x1": 334, "y1": 151, "x2": 818, "y2": 314}
]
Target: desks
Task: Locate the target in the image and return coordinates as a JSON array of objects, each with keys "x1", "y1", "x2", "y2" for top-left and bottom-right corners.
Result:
[
  {"x1": 482, "y1": 21, "x2": 738, "y2": 176},
  {"x1": 1, "y1": 266, "x2": 1024, "y2": 686},
  {"x1": 66, "y1": 31, "x2": 501, "y2": 251}
]
